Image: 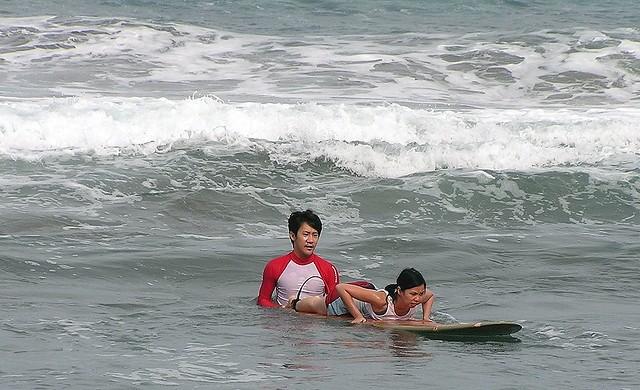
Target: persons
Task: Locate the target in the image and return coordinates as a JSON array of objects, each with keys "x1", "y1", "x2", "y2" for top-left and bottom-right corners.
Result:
[
  {"x1": 256, "y1": 207, "x2": 339, "y2": 308},
  {"x1": 284, "y1": 266, "x2": 444, "y2": 324}
]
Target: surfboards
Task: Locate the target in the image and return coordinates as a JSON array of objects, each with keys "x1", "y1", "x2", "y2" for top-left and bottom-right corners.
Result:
[{"x1": 285, "y1": 309, "x2": 522, "y2": 335}]
